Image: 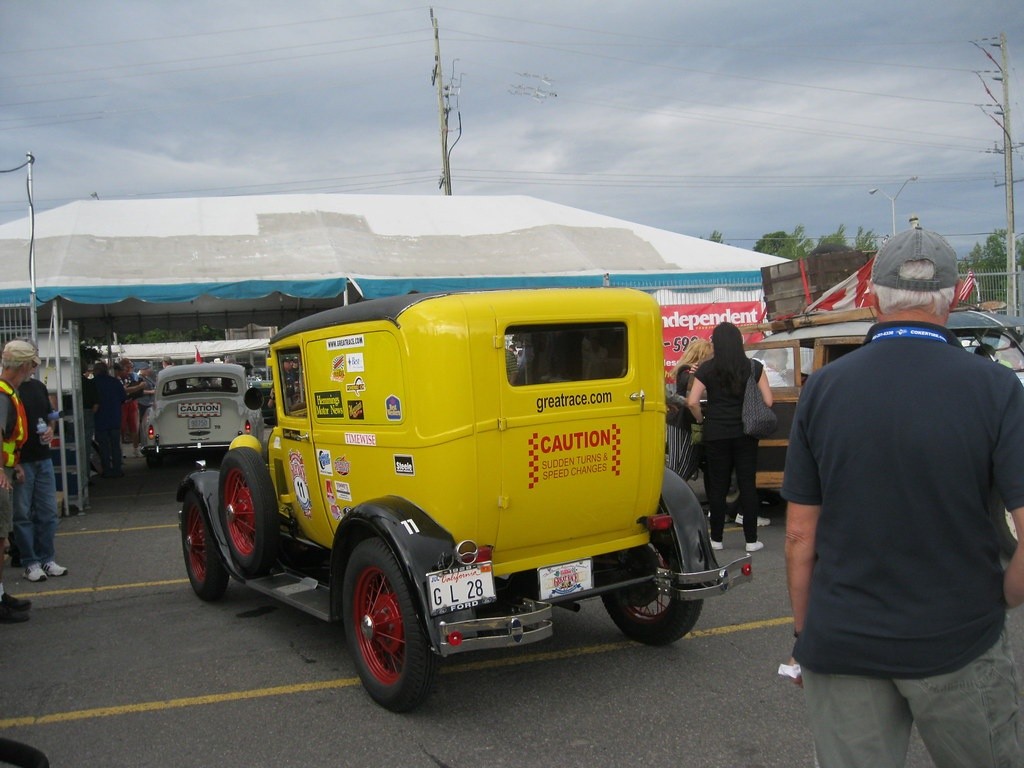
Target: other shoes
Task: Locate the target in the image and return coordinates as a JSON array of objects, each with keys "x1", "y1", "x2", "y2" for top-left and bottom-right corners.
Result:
[
  {"x1": 0, "y1": 606, "x2": 30, "y2": 623},
  {"x1": 0, "y1": 592, "x2": 32, "y2": 612}
]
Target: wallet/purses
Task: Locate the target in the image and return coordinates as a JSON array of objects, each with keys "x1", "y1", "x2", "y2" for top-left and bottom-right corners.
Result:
[{"x1": 690, "y1": 423, "x2": 705, "y2": 446}]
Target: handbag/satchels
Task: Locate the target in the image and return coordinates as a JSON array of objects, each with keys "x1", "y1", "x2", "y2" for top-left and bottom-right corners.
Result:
[{"x1": 741, "y1": 358, "x2": 777, "y2": 439}]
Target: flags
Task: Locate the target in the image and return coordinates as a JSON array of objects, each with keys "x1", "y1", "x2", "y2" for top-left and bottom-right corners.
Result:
[
  {"x1": 959, "y1": 269, "x2": 974, "y2": 301},
  {"x1": 196, "y1": 349, "x2": 202, "y2": 362}
]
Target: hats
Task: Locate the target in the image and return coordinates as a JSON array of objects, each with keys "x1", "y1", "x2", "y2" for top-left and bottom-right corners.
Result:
[
  {"x1": 3, "y1": 340, "x2": 41, "y2": 365},
  {"x1": 871, "y1": 226, "x2": 959, "y2": 290}
]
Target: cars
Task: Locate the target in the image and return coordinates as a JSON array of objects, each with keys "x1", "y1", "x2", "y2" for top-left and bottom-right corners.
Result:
[
  {"x1": 140, "y1": 362, "x2": 266, "y2": 470},
  {"x1": 690, "y1": 310, "x2": 1024, "y2": 491}
]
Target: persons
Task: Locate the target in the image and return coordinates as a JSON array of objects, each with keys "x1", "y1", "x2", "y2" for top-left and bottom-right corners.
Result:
[
  {"x1": 90, "y1": 363, "x2": 127, "y2": 478},
  {"x1": 120, "y1": 358, "x2": 157, "y2": 457},
  {"x1": 666, "y1": 339, "x2": 714, "y2": 482},
  {"x1": 780, "y1": 226, "x2": 1024, "y2": 768},
  {"x1": 0, "y1": 336, "x2": 69, "y2": 622},
  {"x1": 267, "y1": 357, "x2": 296, "y2": 424},
  {"x1": 225, "y1": 357, "x2": 236, "y2": 363},
  {"x1": 114, "y1": 362, "x2": 126, "y2": 389},
  {"x1": 162, "y1": 356, "x2": 177, "y2": 391},
  {"x1": 687, "y1": 321, "x2": 774, "y2": 551},
  {"x1": 81, "y1": 357, "x2": 98, "y2": 480},
  {"x1": 291, "y1": 356, "x2": 298, "y2": 379}
]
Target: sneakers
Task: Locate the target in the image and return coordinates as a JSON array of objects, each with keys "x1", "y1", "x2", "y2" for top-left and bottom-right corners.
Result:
[
  {"x1": 40, "y1": 561, "x2": 68, "y2": 577},
  {"x1": 709, "y1": 537, "x2": 723, "y2": 550},
  {"x1": 22, "y1": 563, "x2": 48, "y2": 582},
  {"x1": 745, "y1": 540, "x2": 764, "y2": 552},
  {"x1": 735, "y1": 513, "x2": 771, "y2": 527}
]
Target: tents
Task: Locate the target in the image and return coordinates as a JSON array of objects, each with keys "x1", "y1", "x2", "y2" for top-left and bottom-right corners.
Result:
[{"x1": 0, "y1": 194, "x2": 793, "y2": 516}]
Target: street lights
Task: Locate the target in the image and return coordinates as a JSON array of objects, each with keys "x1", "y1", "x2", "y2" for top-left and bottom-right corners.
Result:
[{"x1": 869, "y1": 176, "x2": 918, "y2": 235}]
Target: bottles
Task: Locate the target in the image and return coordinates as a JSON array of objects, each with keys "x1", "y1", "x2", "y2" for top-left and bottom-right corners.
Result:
[{"x1": 36, "y1": 417, "x2": 52, "y2": 445}]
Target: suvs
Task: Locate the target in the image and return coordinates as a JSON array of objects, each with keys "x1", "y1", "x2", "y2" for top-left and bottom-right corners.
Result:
[{"x1": 173, "y1": 287, "x2": 753, "y2": 710}]
